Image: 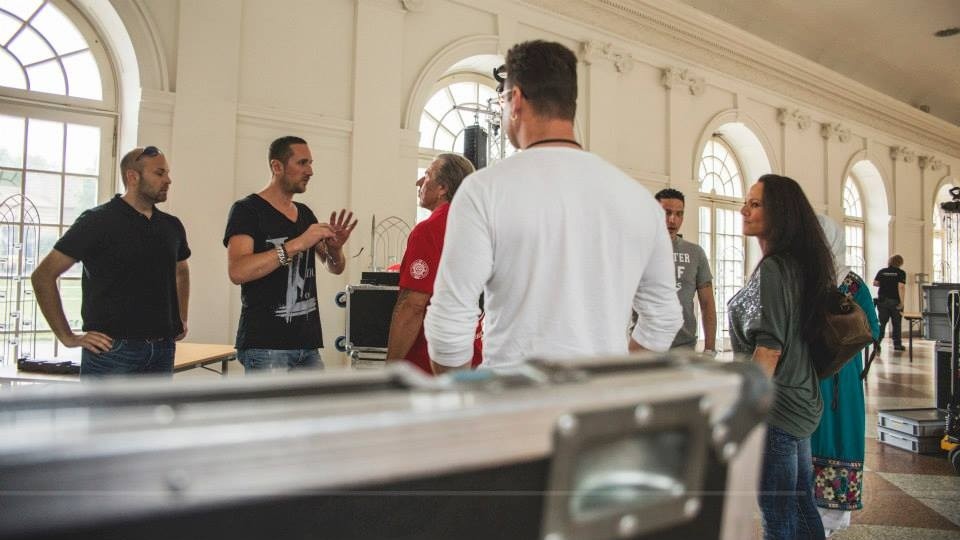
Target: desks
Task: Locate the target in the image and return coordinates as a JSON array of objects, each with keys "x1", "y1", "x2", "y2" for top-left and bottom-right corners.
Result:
[
  {"x1": 900, "y1": 312, "x2": 923, "y2": 362},
  {"x1": 0, "y1": 342, "x2": 239, "y2": 384}
]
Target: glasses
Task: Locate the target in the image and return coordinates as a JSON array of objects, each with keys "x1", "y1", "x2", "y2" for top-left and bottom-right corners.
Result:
[{"x1": 135, "y1": 146, "x2": 160, "y2": 162}]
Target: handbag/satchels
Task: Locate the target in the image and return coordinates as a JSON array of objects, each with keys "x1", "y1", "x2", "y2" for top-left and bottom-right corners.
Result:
[{"x1": 809, "y1": 288, "x2": 871, "y2": 378}]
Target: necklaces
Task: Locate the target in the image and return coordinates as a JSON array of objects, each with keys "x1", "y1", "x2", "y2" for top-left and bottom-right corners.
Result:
[{"x1": 520, "y1": 136, "x2": 581, "y2": 148}]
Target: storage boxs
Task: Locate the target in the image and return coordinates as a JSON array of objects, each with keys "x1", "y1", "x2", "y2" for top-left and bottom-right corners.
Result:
[{"x1": 877, "y1": 282, "x2": 960, "y2": 454}]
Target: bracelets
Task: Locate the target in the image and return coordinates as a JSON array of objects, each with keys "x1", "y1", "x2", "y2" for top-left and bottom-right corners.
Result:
[{"x1": 275, "y1": 242, "x2": 292, "y2": 267}]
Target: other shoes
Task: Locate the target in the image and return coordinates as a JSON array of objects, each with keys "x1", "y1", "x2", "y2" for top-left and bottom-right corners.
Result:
[{"x1": 894, "y1": 346, "x2": 905, "y2": 350}]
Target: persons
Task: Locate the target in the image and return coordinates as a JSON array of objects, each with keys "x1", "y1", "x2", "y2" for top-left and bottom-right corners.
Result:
[
  {"x1": 873, "y1": 255, "x2": 907, "y2": 355},
  {"x1": 222, "y1": 135, "x2": 359, "y2": 375},
  {"x1": 384, "y1": 153, "x2": 485, "y2": 376},
  {"x1": 424, "y1": 39, "x2": 683, "y2": 379},
  {"x1": 807, "y1": 213, "x2": 879, "y2": 538},
  {"x1": 29, "y1": 146, "x2": 190, "y2": 375},
  {"x1": 728, "y1": 173, "x2": 834, "y2": 540},
  {"x1": 631, "y1": 187, "x2": 718, "y2": 355}
]
